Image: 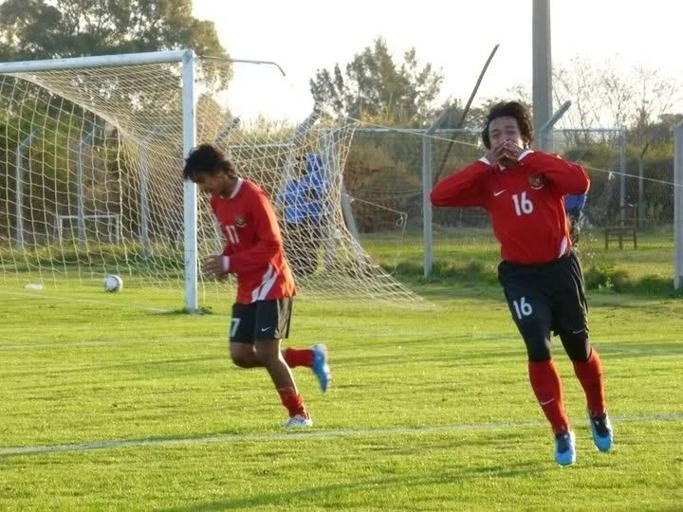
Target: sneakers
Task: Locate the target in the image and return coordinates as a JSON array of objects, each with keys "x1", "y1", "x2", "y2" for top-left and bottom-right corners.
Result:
[
  {"x1": 285, "y1": 412, "x2": 313, "y2": 429},
  {"x1": 588, "y1": 411, "x2": 613, "y2": 451},
  {"x1": 554, "y1": 428, "x2": 576, "y2": 466},
  {"x1": 310, "y1": 344, "x2": 329, "y2": 392}
]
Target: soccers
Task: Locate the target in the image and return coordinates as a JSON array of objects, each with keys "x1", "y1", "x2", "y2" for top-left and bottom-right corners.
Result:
[{"x1": 104, "y1": 274, "x2": 122, "y2": 293}]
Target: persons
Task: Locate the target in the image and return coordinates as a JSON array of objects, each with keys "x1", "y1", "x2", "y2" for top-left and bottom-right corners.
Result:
[
  {"x1": 565, "y1": 194, "x2": 587, "y2": 245},
  {"x1": 183, "y1": 142, "x2": 329, "y2": 432},
  {"x1": 283, "y1": 152, "x2": 326, "y2": 276},
  {"x1": 429, "y1": 100, "x2": 615, "y2": 464}
]
